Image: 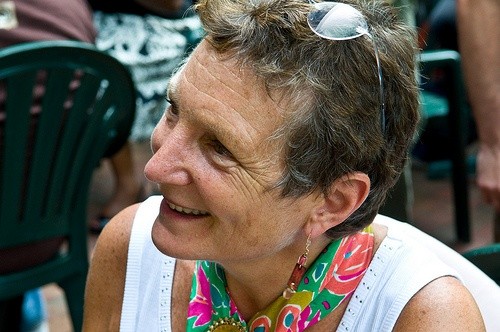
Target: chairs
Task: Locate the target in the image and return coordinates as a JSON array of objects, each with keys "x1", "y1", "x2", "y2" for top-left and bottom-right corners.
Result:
[
  {"x1": 0, "y1": 37, "x2": 137, "y2": 332},
  {"x1": 396, "y1": 49, "x2": 482, "y2": 246}
]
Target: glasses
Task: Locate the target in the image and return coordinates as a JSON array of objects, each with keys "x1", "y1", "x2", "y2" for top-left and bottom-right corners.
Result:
[{"x1": 305, "y1": 1, "x2": 386, "y2": 133}]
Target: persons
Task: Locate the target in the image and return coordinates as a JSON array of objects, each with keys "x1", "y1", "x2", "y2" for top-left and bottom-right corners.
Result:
[
  {"x1": 456, "y1": 0, "x2": 500, "y2": 243},
  {"x1": 81, "y1": 0, "x2": 485, "y2": 332},
  {"x1": 0, "y1": 1, "x2": 97, "y2": 332},
  {"x1": 89, "y1": 0, "x2": 206, "y2": 231}
]
